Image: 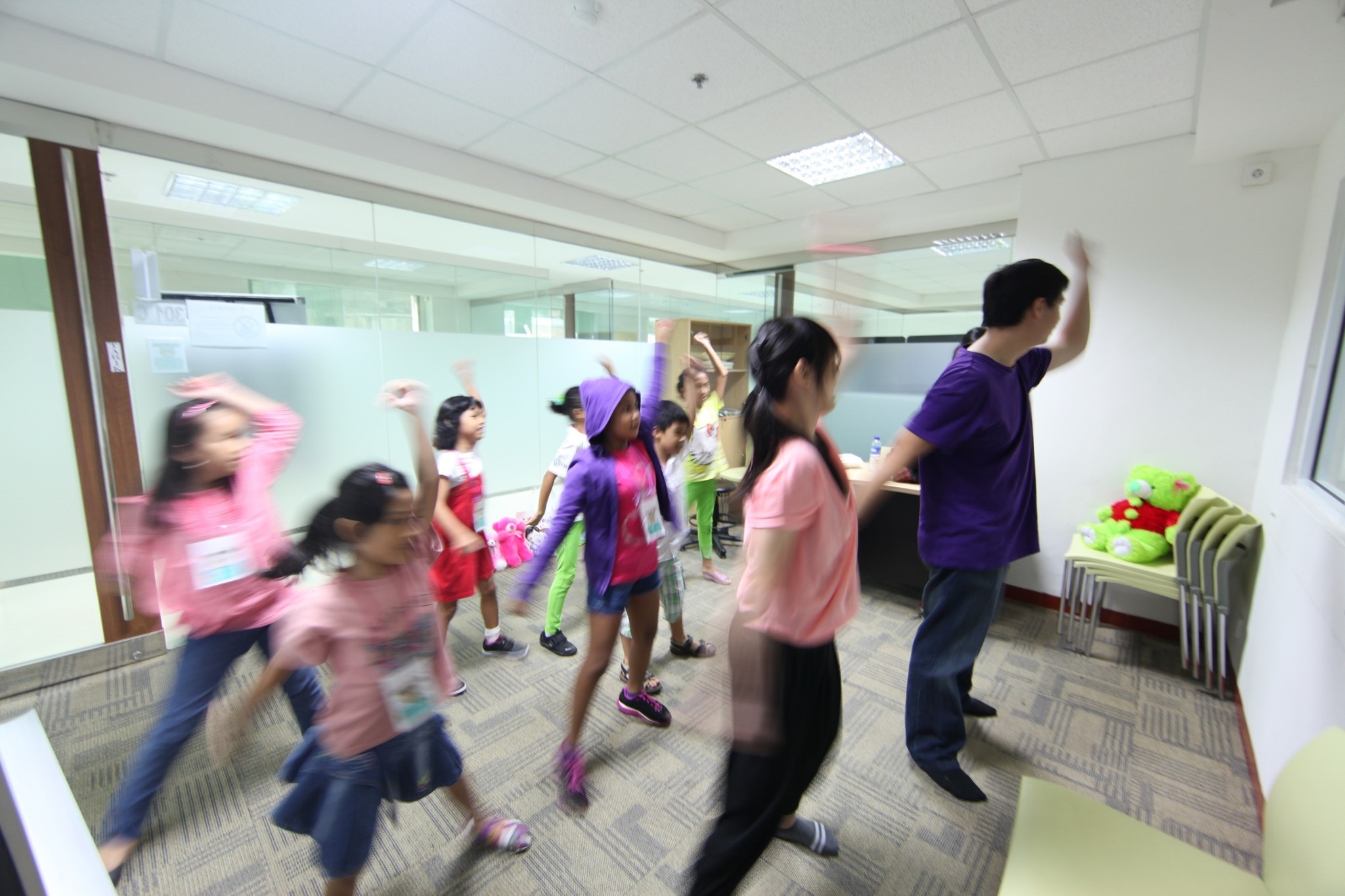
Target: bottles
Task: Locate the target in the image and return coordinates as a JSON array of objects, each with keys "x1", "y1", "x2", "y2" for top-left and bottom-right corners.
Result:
[{"x1": 869, "y1": 436, "x2": 882, "y2": 472}]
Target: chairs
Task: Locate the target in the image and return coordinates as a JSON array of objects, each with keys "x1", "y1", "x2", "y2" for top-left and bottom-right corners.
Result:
[
  {"x1": 1056, "y1": 486, "x2": 1265, "y2": 700},
  {"x1": 995, "y1": 721, "x2": 1345, "y2": 895}
]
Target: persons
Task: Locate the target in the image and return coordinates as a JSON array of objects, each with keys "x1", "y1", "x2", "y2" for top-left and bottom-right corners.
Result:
[
  {"x1": 681, "y1": 199, "x2": 878, "y2": 896},
  {"x1": 523, "y1": 360, "x2": 622, "y2": 654},
  {"x1": 616, "y1": 354, "x2": 702, "y2": 695},
  {"x1": 850, "y1": 228, "x2": 1092, "y2": 803},
  {"x1": 671, "y1": 329, "x2": 731, "y2": 586},
  {"x1": 95, "y1": 372, "x2": 331, "y2": 886},
  {"x1": 508, "y1": 316, "x2": 687, "y2": 811},
  {"x1": 231, "y1": 381, "x2": 532, "y2": 893},
  {"x1": 424, "y1": 358, "x2": 530, "y2": 691}
]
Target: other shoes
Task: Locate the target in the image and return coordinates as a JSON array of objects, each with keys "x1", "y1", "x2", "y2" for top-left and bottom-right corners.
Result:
[{"x1": 470, "y1": 816, "x2": 532, "y2": 854}]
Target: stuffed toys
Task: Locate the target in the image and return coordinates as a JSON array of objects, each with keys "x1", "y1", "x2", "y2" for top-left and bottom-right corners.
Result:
[{"x1": 1079, "y1": 464, "x2": 1198, "y2": 562}]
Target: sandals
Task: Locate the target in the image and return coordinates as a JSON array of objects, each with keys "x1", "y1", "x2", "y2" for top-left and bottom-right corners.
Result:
[
  {"x1": 619, "y1": 657, "x2": 663, "y2": 695},
  {"x1": 670, "y1": 634, "x2": 717, "y2": 658}
]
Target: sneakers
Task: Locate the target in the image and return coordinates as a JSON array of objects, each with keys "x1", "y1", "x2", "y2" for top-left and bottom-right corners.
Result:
[
  {"x1": 539, "y1": 629, "x2": 577, "y2": 656},
  {"x1": 449, "y1": 679, "x2": 467, "y2": 696},
  {"x1": 481, "y1": 634, "x2": 530, "y2": 659},
  {"x1": 557, "y1": 738, "x2": 589, "y2": 808},
  {"x1": 616, "y1": 685, "x2": 672, "y2": 727}
]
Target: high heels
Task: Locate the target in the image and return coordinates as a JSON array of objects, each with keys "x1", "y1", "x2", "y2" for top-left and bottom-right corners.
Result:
[{"x1": 702, "y1": 569, "x2": 731, "y2": 584}]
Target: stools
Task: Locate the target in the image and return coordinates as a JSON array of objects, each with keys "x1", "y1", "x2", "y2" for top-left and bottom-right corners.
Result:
[{"x1": 678, "y1": 477, "x2": 748, "y2": 558}]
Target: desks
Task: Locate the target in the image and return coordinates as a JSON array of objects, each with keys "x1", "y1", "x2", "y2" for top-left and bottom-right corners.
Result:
[{"x1": 838, "y1": 457, "x2": 922, "y2": 498}]
